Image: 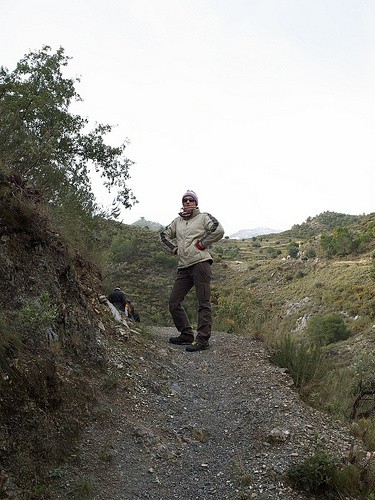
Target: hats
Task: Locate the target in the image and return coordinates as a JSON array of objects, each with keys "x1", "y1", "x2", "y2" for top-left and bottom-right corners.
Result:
[{"x1": 182, "y1": 190, "x2": 198, "y2": 206}]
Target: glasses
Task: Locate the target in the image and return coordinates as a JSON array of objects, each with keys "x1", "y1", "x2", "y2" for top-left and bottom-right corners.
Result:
[{"x1": 183, "y1": 198, "x2": 195, "y2": 203}]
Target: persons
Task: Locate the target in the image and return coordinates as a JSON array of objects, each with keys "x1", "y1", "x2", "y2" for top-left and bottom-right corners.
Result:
[
  {"x1": 159, "y1": 189, "x2": 225, "y2": 352},
  {"x1": 108, "y1": 285, "x2": 126, "y2": 312},
  {"x1": 128, "y1": 304, "x2": 140, "y2": 322}
]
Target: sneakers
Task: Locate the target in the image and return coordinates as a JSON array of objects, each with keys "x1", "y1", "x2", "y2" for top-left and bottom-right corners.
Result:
[
  {"x1": 186, "y1": 340, "x2": 211, "y2": 352},
  {"x1": 169, "y1": 336, "x2": 194, "y2": 345}
]
